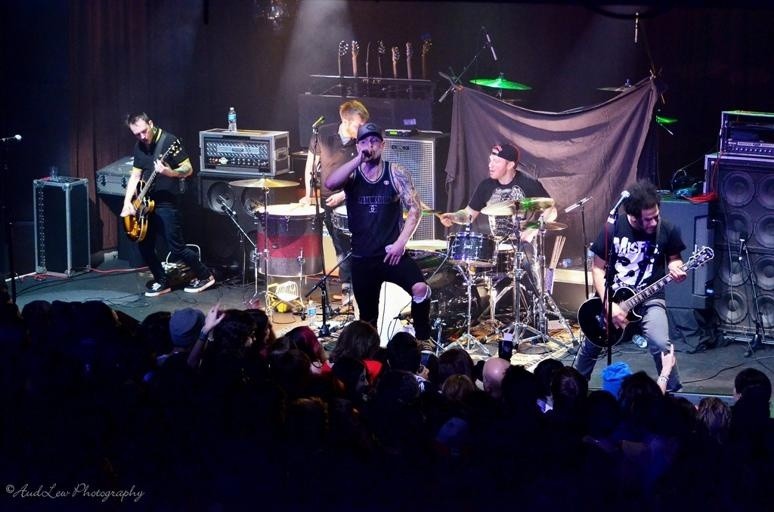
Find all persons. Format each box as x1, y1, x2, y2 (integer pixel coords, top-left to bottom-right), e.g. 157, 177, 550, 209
312, 319, 594, 512
325, 123, 434, 351
120, 110, 216, 297
297, 101, 369, 302
591, 358, 774, 510
0, 283, 324, 512
573, 181, 682, 394
439, 144, 558, 343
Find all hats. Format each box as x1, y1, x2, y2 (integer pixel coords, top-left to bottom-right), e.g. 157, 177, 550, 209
490, 142, 528, 169
170, 305, 206, 346
357, 122, 383, 144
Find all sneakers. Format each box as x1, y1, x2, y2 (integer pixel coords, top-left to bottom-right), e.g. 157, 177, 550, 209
416, 338, 436, 354
184, 275, 216, 293
340, 282, 355, 306
667, 384, 685, 393
144, 279, 172, 297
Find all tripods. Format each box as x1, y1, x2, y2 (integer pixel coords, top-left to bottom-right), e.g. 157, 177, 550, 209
494, 271, 577, 356
437, 286, 493, 357
248, 194, 302, 322
306, 254, 350, 339
510, 234, 581, 350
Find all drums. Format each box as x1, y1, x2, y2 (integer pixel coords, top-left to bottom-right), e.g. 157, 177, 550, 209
330, 205, 352, 259
447, 232, 497, 268
256, 205, 327, 278
476, 243, 518, 274
404, 249, 481, 354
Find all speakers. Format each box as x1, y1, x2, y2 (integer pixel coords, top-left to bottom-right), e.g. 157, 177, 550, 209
198, 171, 270, 277
702, 155, 774, 344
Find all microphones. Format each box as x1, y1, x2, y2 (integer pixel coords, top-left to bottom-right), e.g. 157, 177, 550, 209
362, 149, 373, 158
216, 194, 236, 218
1, 133, 23, 143
609, 190, 630, 215
312, 115, 327, 129
736, 230, 748, 260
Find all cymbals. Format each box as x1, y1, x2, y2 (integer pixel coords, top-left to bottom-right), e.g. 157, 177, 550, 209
403, 207, 437, 217
520, 221, 568, 232
481, 196, 555, 217
469, 72, 533, 91
596, 79, 634, 92
229, 177, 299, 190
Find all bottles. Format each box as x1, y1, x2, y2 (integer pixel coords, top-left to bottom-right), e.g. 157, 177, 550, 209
633, 334, 648, 348
555, 256, 581, 268
306, 300, 318, 326
587, 242, 596, 271
227, 107, 237, 132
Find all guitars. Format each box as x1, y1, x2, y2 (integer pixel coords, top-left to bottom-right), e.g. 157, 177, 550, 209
577, 247, 716, 348
349, 39, 362, 98
374, 39, 392, 99
402, 42, 414, 99
121, 138, 183, 243
391, 46, 403, 99
333, 40, 350, 96
418, 38, 435, 98
363, 40, 374, 97
